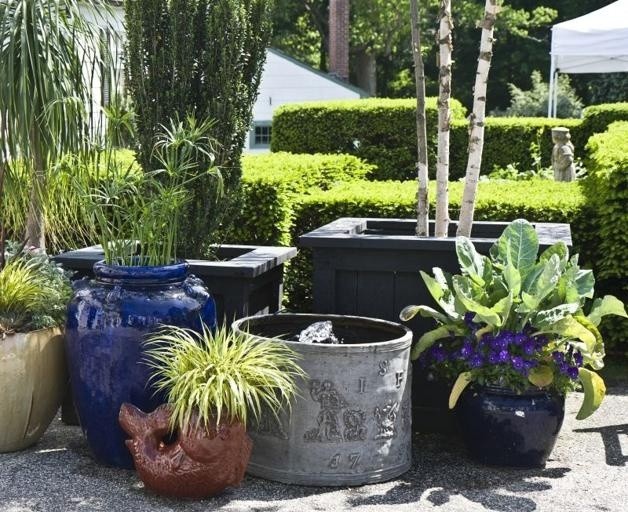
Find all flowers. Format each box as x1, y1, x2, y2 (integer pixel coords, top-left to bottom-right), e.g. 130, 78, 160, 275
392, 218, 628, 421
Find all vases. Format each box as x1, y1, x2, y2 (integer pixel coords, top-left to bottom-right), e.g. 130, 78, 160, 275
472, 382, 565, 468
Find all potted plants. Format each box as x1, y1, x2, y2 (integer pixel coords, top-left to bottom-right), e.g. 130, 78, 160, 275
0, 0, 301, 499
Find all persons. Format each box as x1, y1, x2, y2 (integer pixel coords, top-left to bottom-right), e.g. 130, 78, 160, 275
551, 128, 575, 182
